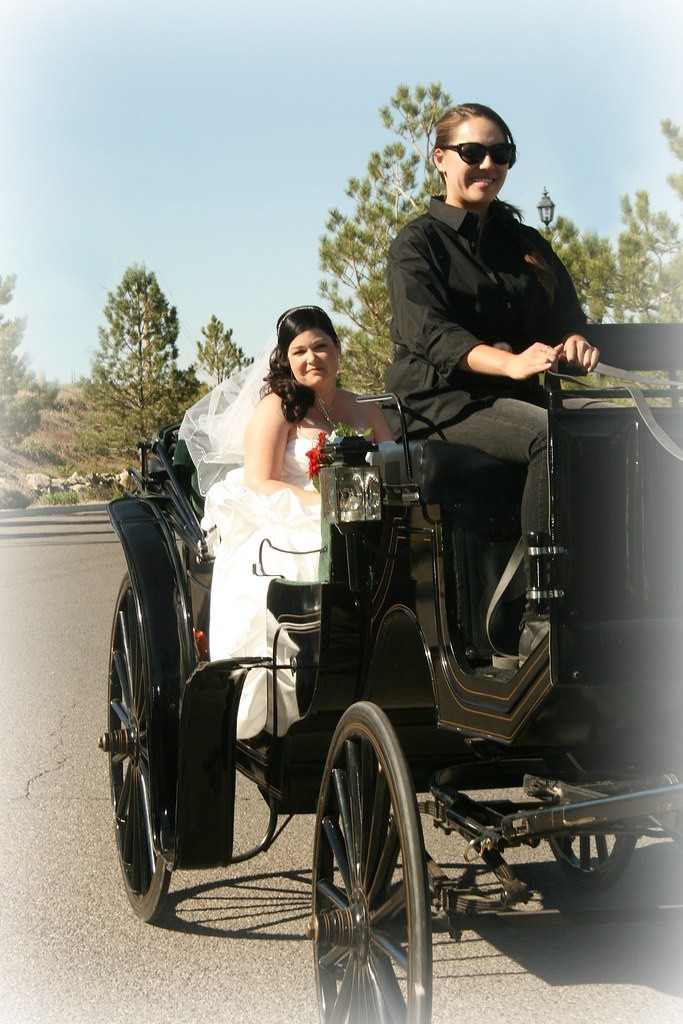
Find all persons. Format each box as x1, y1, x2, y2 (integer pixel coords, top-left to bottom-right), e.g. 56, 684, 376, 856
176, 304, 397, 739
388, 104, 599, 668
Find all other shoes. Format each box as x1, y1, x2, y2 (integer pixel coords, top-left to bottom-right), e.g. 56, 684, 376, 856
518, 622, 551, 669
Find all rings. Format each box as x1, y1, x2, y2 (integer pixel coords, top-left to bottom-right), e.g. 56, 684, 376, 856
545, 345, 550, 353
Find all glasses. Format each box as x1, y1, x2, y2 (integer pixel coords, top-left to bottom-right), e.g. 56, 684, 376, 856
438, 143, 516, 166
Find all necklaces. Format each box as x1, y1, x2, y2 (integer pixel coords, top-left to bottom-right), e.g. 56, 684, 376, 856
314, 402, 336, 422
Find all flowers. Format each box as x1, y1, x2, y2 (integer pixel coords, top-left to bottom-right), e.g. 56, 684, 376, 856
305, 421, 374, 494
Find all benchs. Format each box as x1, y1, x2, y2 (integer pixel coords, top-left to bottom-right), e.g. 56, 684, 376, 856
354, 324, 681, 663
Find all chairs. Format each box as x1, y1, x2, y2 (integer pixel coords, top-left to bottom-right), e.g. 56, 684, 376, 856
148, 422, 218, 662
